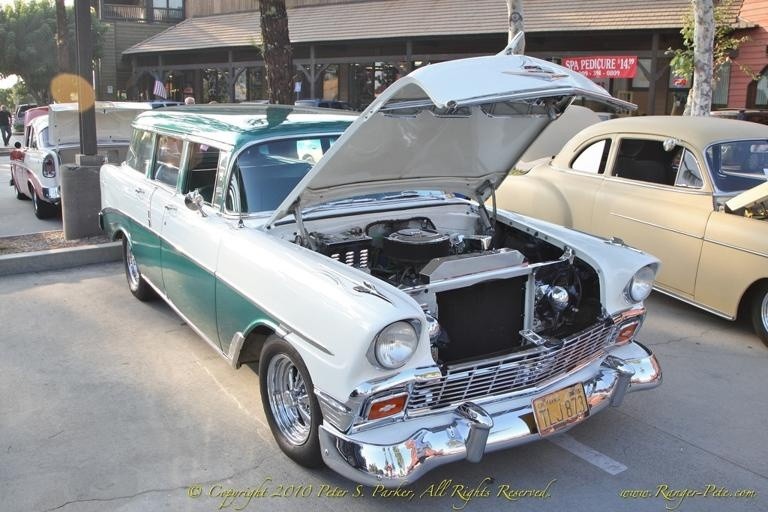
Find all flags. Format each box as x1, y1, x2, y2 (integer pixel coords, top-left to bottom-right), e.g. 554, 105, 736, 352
152, 79, 167, 100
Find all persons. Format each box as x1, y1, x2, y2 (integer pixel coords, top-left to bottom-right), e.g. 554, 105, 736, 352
185, 96, 195, 105
0, 104, 14, 146
669, 93, 685, 115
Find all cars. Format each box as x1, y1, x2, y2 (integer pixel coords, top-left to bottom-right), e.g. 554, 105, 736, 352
98, 27, 665, 491
477, 101, 768, 346
9, 97, 366, 220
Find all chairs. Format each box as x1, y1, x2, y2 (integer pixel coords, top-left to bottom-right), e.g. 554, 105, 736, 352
615, 141, 666, 182
188, 166, 218, 205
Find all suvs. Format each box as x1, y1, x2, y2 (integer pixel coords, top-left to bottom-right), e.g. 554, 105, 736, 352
706, 108, 768, 174
